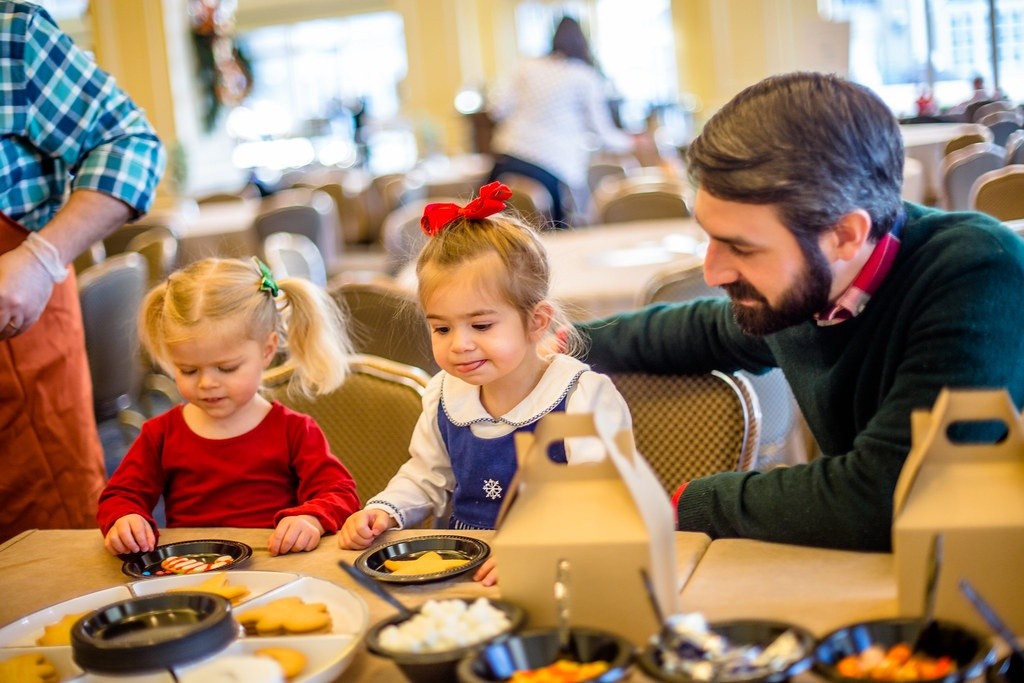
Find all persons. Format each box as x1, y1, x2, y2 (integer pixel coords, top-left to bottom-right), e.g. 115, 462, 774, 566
970, 77, 989, 101
529, 72, 1024, 554
340, 183, 640, 585
92, 257, 359, 554
489, 16, 654, 224
0, 0, 166, 530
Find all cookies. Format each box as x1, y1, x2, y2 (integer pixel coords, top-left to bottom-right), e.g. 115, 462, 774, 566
384, 550, 470, 576
507, 659, 609, 683
160, 556, 235, 573
0, 611, 95, 683
236, 596, 336, 678
165, 573, 250, 601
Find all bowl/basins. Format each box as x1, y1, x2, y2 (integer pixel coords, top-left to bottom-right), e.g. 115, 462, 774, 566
454, 624, 637, 682
806, 616, 994, 682
368, 590, 530, 682
634, 616, 822, 682
983, 639, 1024, 682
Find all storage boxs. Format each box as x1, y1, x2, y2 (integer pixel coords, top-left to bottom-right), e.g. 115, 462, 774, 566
890, 385, 1024, 642
490, 410, 668, 653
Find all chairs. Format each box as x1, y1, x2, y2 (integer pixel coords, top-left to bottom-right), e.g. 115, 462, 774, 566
72, 99, 1024, 529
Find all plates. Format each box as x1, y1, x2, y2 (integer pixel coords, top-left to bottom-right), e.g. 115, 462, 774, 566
355, 533, 492, 585
122, 539, 253, 579
0, 567, 369, 682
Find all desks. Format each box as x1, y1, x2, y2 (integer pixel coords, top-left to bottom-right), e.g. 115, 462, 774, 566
677, 536, 896, 639
534, 214, 708, 327
897, 122, 995, 201
0, 526, 715, 683
174, 197, 260, 268
